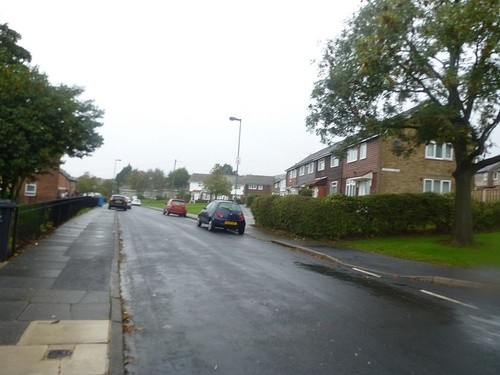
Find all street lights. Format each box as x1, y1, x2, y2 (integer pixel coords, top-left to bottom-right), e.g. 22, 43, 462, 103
230, 116, 242, 199
113, 159, 121, 194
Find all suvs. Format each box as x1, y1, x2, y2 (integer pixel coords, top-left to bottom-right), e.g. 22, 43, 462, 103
109, 195, 128, 211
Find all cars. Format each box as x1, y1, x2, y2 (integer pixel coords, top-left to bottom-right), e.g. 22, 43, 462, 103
197, 199, 246, 235
126, 196, 141, 209
163, 199, 187, 218
